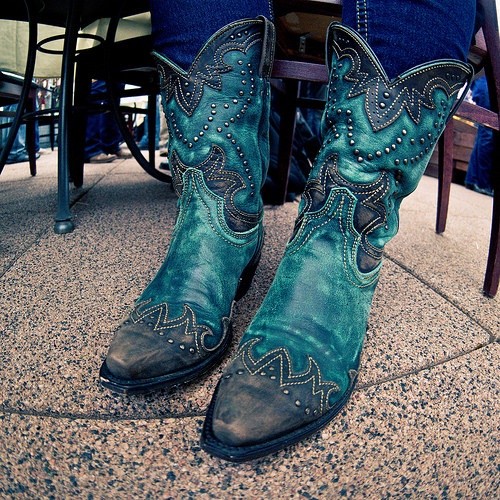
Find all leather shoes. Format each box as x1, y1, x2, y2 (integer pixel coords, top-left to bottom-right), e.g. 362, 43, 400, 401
6, 153, 41, 164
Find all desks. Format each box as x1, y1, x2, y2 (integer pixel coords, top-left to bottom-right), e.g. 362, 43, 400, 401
0, 0, 500, 301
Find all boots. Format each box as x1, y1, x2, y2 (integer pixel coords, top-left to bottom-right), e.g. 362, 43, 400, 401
97, 14, 279, 395
199, 23, 475, 463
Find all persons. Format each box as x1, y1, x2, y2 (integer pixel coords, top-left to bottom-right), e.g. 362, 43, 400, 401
98, 0, 478, 462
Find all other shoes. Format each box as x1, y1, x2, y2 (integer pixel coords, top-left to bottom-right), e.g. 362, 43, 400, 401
474, 185, 493, 196
464, 182, 474, 190
135, 135, 169, 170
87, 152, 117, 164
112, 149, 132, 159
